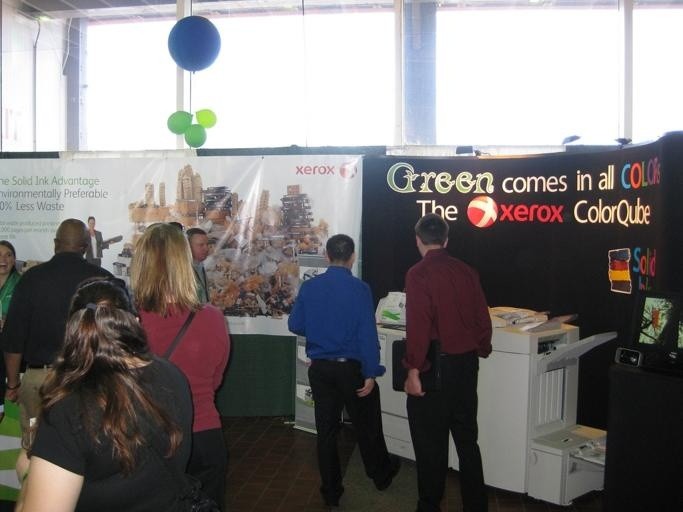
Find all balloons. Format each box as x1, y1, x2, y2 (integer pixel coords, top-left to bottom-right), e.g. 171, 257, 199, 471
168, 15, 221, 75
167, 111, 193, 134
196, 109, 217, 128
184, 124, 206, 148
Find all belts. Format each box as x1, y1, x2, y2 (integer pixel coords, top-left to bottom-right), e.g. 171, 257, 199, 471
28, 363, 53, 368
327, 357, 347, 362
440, 353, 464, 360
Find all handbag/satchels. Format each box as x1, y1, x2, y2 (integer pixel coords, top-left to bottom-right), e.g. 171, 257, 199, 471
169, 485, 219, 512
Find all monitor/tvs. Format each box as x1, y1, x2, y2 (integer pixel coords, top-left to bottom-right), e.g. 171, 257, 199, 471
630, 288, 682, 377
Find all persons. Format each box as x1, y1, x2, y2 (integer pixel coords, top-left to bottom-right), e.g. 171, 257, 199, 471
401, 212, 488, 511
288, 234, 392, 511
1, 218, 231, 511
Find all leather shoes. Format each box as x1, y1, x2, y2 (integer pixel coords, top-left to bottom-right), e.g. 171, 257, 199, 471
325, 486, 344, 505
374, 454, 399, 490
